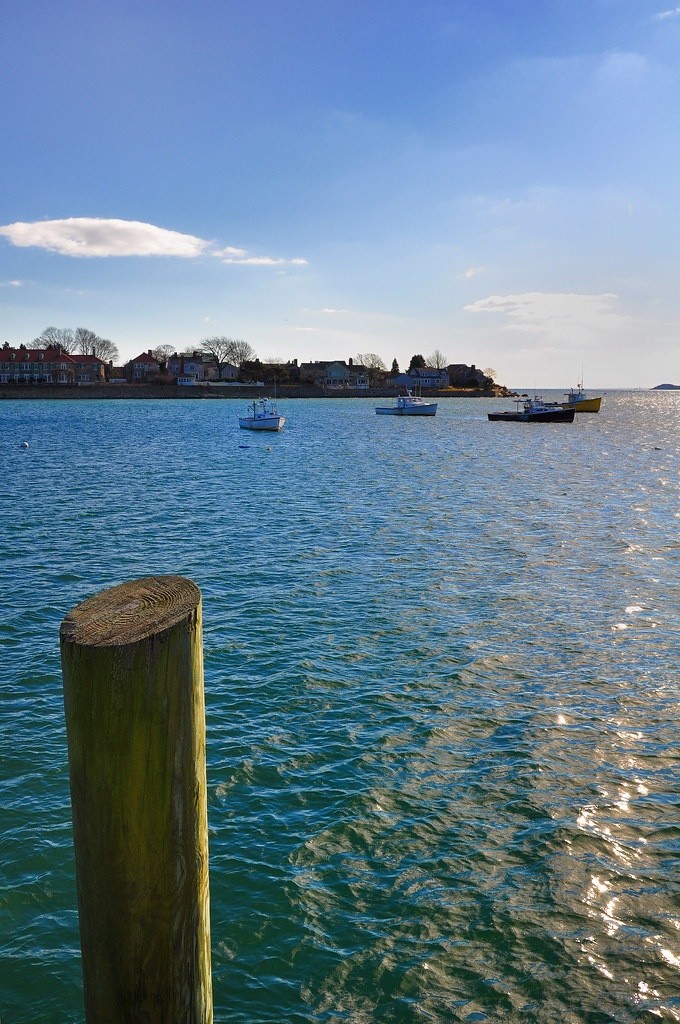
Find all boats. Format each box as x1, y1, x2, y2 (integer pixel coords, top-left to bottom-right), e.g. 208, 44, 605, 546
238, 379, 285, 431
486, 363, 602, 423
374, 380, 438, 417
